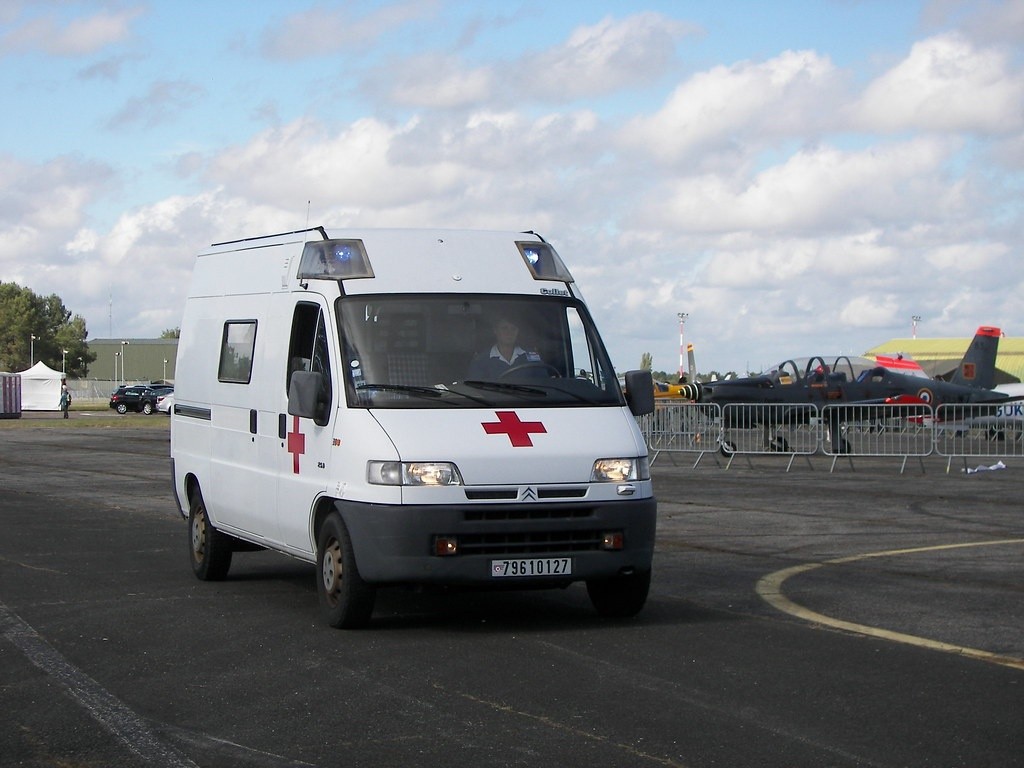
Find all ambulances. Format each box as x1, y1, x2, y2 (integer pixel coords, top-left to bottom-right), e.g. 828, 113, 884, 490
169, 223, 660, 629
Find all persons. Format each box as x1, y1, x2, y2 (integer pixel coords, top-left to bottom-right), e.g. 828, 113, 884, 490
467, 313, 563, 381
803, 365, 830, 387
58, 385, 72, 419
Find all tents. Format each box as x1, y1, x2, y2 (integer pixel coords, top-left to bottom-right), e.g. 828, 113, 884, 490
15, 360, 67, 411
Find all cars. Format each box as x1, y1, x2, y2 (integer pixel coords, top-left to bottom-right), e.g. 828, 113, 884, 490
154, 391, 174, 415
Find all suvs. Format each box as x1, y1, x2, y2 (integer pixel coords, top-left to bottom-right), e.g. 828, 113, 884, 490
109, 383, 174, 415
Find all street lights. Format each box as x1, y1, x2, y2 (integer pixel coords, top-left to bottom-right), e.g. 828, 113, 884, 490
30, 333, 40, 368
114, 351, 121, 388
163, 358, 168, 384
677, 312, 689, 382
120, 340, 130, 386
62, 349, 69, 373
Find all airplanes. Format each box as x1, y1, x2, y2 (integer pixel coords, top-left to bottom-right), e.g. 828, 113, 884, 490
863, 352, 1024, 441
652, 325, 1010, 457
596, 343, 704, 404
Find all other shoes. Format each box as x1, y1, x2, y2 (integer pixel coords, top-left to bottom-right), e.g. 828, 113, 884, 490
64, 416, 68, 419
